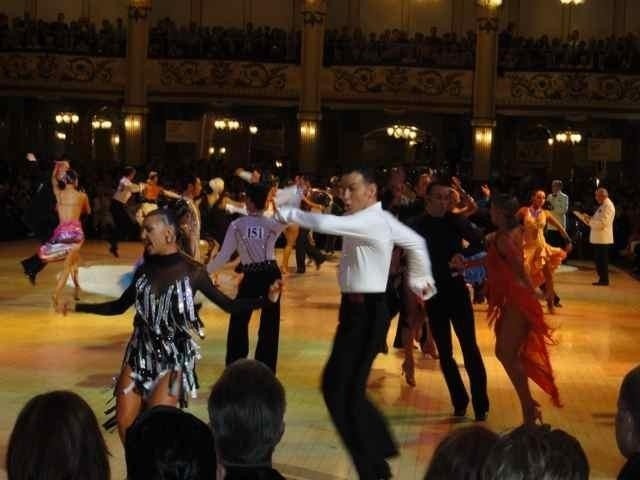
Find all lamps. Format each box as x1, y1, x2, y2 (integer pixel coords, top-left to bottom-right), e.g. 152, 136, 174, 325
53, 114, 80, 122
91, 118, 112, 129
548, 130, 581, 146
386, 125, 421, 140
214, 117, 238, 130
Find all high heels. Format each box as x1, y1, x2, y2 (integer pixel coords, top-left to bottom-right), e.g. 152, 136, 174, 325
423, 344, 440, 360
524, 400, 544, 426
402, 362, 416, 386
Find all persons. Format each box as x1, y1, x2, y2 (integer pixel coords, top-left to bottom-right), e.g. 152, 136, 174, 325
276, 158, 436, 479
7, 359, 287, 479
109, 166, 346, 273
425, 366, 639, 479
57, 207, 283, 451
0, 9, 127, 57
324, 22, 477, 70
381, 165, 615, 424
148, 16, 302, 64
497, 20, 640, 75
204, 186, 288, 374
21, 158, 92, 309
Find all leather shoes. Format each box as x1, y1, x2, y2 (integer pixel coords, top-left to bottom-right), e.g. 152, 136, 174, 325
454, 399, 488, 421
